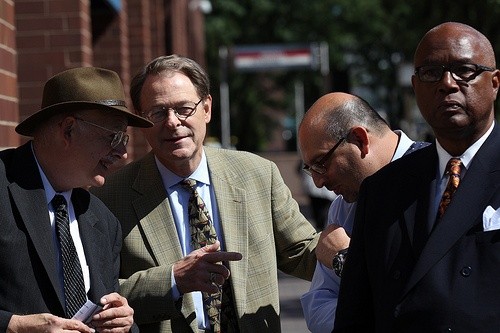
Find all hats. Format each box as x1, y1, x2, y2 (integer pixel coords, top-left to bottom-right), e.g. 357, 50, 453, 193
14, 66, 155, 139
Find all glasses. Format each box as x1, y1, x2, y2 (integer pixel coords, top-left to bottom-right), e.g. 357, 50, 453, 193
413, 61, 495, 84
139, 96, 204, 126
57, 115, 130, 150
302, 126, 369, 178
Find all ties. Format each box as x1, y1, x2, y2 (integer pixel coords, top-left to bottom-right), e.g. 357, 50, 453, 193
180, 179, 231, 333
50, 194, 88, 320
433, 157, 463, 229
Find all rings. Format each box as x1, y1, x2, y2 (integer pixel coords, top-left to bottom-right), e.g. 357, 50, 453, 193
210, 272, 217, 282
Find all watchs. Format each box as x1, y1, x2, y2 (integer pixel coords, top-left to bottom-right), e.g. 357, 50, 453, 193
332, 247, 349, 278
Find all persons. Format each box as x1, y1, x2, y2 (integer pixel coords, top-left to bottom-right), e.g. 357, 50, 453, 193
89, 53, 323, 333
331, 22, 500, 333
0, 66, 155, 333
297, 92, 432, 333
